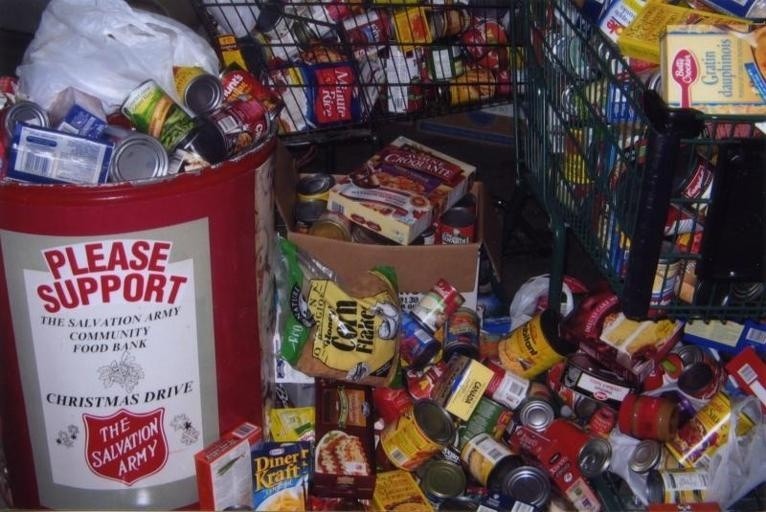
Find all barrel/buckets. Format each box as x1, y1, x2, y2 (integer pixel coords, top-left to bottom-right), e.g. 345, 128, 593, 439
0, 97, 281, 510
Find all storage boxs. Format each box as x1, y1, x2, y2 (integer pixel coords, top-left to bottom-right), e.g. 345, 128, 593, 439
617, 1, 765, 117
268, 140, 505, 389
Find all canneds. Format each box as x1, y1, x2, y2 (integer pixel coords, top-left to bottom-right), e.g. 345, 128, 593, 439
0, 0, 766, 512
